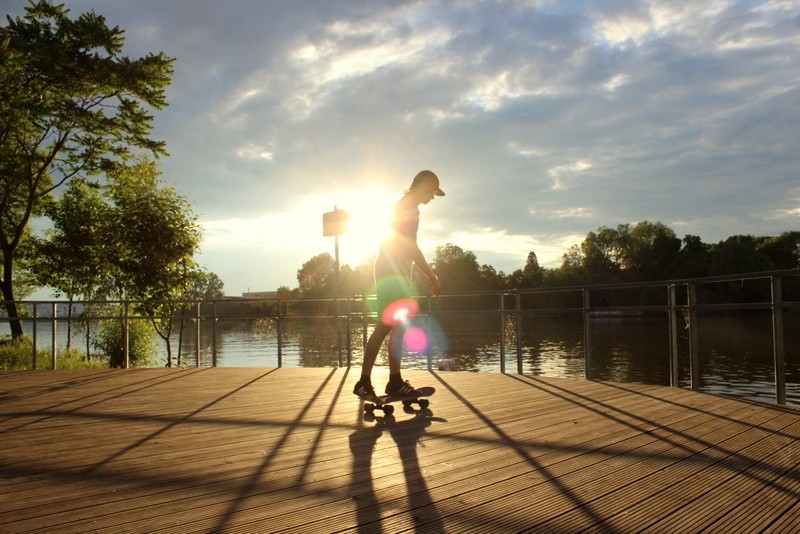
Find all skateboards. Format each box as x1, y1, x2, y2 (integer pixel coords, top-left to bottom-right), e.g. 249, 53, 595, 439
357, 387, 435, 415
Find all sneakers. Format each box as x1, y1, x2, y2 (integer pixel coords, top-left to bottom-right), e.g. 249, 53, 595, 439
385, 379, 424, 396
353, 381, 383, 403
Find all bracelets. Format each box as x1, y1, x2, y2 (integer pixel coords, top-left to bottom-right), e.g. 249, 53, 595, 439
430, 275, 438, 283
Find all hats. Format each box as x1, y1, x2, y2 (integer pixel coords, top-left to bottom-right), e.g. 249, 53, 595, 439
414, 170, 445, 196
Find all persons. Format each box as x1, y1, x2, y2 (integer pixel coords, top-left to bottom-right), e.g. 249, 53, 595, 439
353, 170, 446, 402
